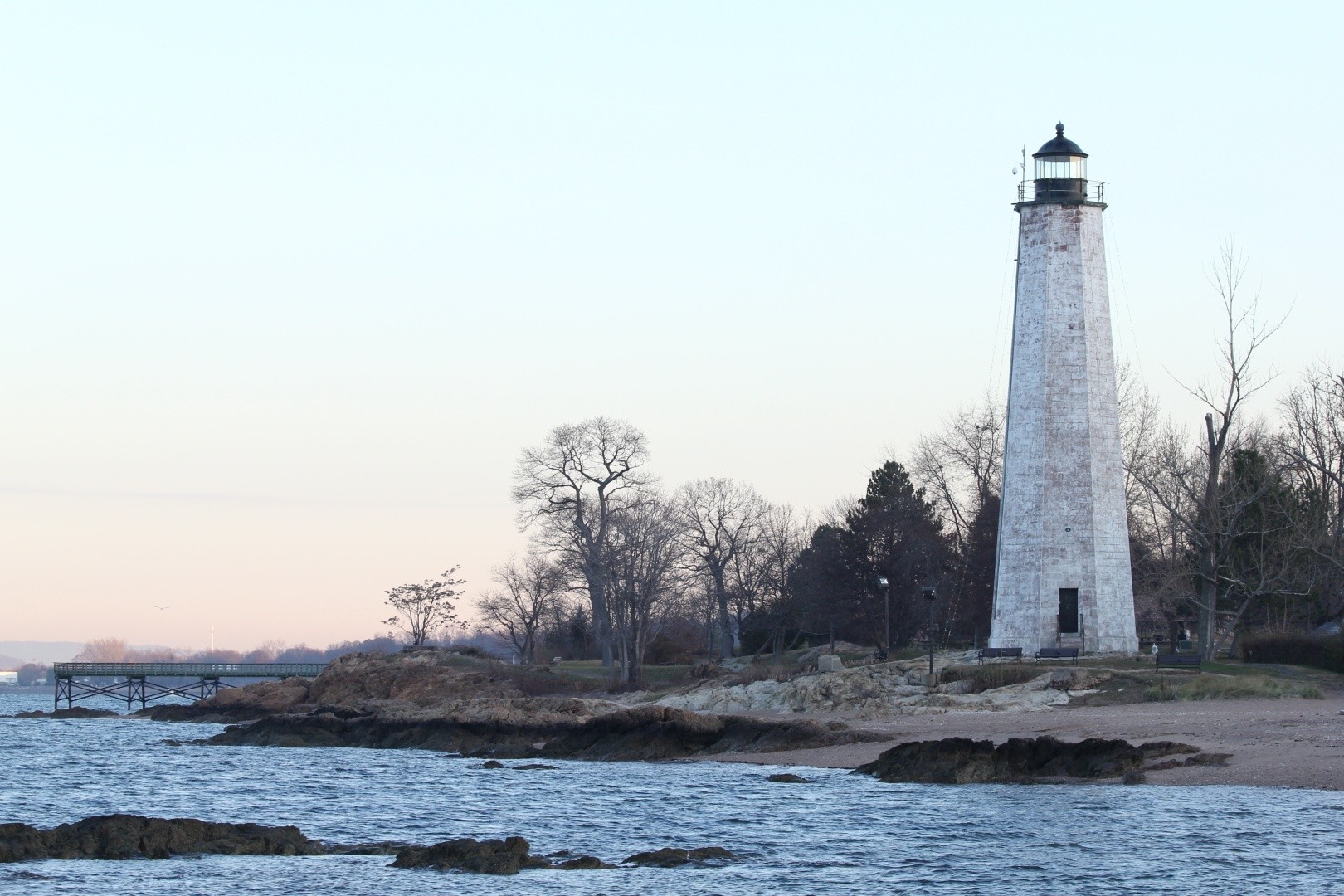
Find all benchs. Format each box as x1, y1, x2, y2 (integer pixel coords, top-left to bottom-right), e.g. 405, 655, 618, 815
873, 650, 888, 663
978, 647, 1023, 664
1156, 654, 1203, 674
660, 657, 678, 666
1035, 647, 1079, 666
1177, 641, 1194, 654
1219, 648, 1230, 654
550, 657, 562, 663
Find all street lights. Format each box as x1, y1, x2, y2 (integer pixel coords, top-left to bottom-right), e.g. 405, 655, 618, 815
921, 586, 937, 687
879, 577, 890, 662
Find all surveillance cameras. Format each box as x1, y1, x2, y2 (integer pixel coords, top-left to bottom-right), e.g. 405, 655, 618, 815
1012, 168, 1017, 174
